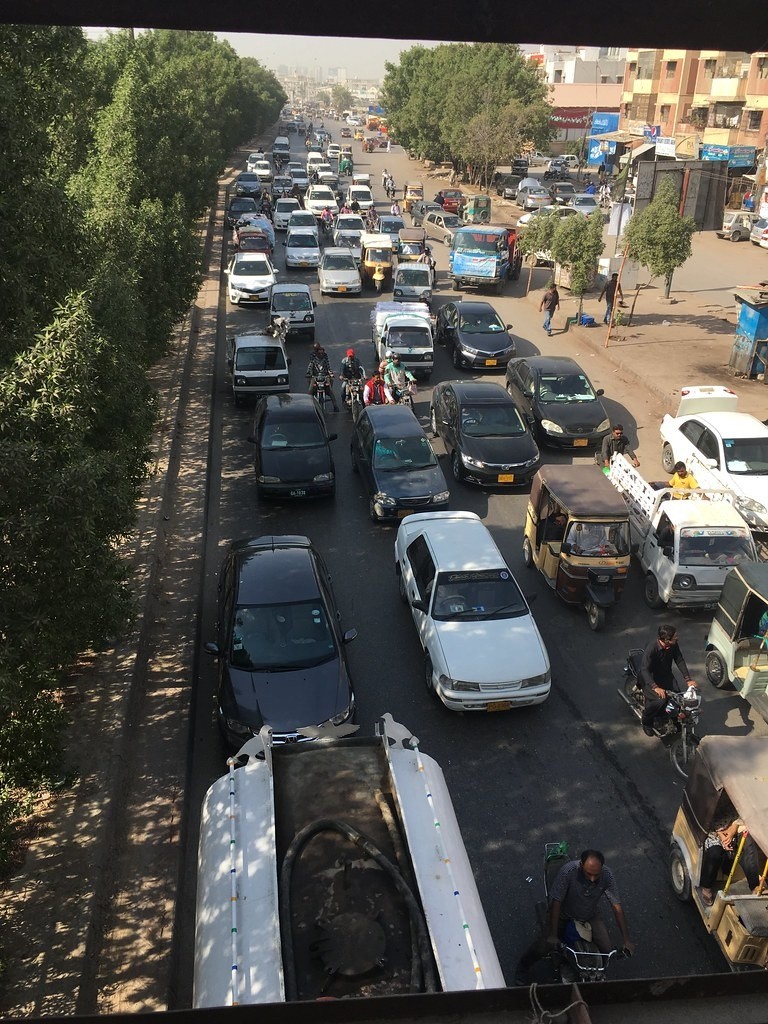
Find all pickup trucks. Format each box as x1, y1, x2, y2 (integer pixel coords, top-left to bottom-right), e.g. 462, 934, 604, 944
369, 302, 435, 381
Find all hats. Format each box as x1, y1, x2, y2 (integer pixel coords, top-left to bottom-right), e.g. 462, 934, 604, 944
347, 349, 353, 357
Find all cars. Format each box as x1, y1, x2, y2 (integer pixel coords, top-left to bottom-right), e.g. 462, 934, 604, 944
351, 405, 451, 521
247, 393, 338, 502
716, 212, 768, 249
394, 511, 552, 713
224, 106, 602, 405
430, 379, 540, 486
216, 535, 356, 759
661, 385, 768, 533
505, 355, 610, 450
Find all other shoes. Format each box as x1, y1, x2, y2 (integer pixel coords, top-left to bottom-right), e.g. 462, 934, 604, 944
644, 724, 654, 736
543, 325, 546, 330
700, 887, 714, 905
547, 331, 551, 336
752, 885, 768, 895
334, 407, 339, 412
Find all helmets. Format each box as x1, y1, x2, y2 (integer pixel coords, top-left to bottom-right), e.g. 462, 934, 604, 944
391, 352, 401, 360
386, 351, 392, 358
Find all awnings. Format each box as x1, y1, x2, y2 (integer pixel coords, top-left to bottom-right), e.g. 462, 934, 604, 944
619, 143, 655, 164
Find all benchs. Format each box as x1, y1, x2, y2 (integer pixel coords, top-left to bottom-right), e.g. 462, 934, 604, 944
543, 542, 561, 580
734, 637, 768, 667
716, 899, 768, 964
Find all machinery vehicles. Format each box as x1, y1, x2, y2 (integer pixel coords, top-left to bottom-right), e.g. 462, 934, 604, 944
193, 713, 507, 1005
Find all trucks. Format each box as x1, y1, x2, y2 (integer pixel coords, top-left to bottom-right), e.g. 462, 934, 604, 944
447, 223, 523, 297
607, 453, 759, 611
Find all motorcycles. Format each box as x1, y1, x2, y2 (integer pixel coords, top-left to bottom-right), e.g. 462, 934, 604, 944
310, 375, 417, 422
383, 177, 396, 202
618, 649, 703, 780
457, 194, 492, 224
536, 840, 631, 981
524, 465, 641, 632
544, 169, 560, 182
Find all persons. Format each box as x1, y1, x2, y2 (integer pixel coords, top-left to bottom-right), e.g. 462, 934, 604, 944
258, 134, 355, 183
433, 187, 474, 219
292, 183, 301, 198
640, 624, 698, 737
260, 188, 272, 206
664, 461, 710, 500
657, 523, 676, 556
321, 205, 335, 234
700, 795, 768, 905
539, 283, 562, 336
425, 577, 450, 598
566, 522, 608, 556
547, 849, 637, 982
599, 272, 624, 328
382, 169, 396, 197
340, 198, 381, 227
601, 423, 640, 469
416, 247, 436, 289
309, 347, 417, 413
392, 200, 401, 217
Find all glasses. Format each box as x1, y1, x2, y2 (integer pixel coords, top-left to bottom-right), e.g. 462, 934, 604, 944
614, 432, 622, 435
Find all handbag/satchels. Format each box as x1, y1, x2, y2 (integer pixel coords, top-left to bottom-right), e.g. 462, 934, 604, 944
684, 685, 701, 725
406, 380, 417, 396
